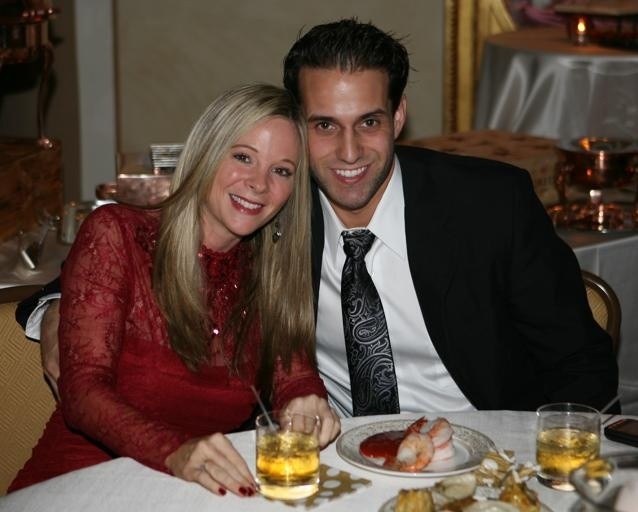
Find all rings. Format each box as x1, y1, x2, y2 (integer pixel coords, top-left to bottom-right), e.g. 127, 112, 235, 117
313, 415, 322, 423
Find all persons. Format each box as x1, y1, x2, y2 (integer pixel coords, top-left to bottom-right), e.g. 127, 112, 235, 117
5, 84, 342, 495
283, 21, 622, 419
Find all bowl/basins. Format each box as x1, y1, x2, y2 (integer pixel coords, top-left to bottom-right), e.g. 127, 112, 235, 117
568, 450, 637, 512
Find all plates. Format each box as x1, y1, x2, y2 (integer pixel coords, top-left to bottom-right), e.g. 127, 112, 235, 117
336, 420, 495, 477
378, 484, 551, 511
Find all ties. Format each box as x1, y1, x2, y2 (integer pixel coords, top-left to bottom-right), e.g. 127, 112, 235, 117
341, 230, 400, 415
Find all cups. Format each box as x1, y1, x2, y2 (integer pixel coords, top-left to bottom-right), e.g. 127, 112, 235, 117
255, 411, 321, 499
533, 401, 602, 495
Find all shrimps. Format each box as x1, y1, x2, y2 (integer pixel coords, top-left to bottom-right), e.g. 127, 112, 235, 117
396, 432, 433, 472
404, 415, 455, 461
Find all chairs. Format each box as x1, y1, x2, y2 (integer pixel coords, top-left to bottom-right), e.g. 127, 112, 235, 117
582, 270, 621, 342
1, 284, 58, 495
